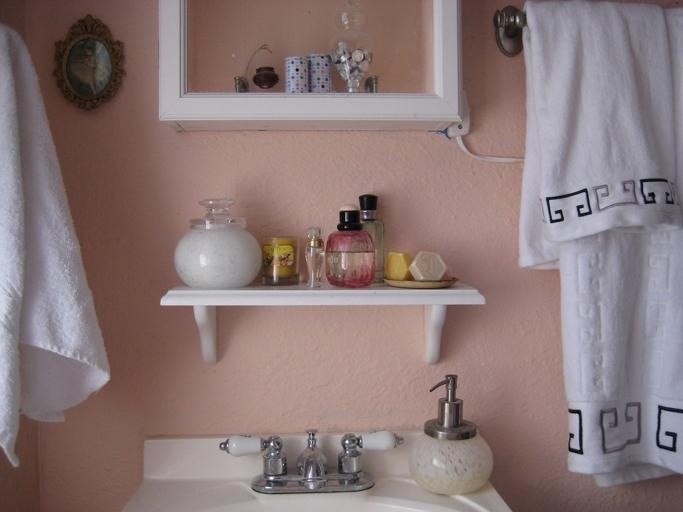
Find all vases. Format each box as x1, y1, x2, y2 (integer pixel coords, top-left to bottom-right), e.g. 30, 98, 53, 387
175, 199, 264, 288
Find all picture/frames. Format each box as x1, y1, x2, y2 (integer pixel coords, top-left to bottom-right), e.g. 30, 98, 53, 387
50, 12, 127, 112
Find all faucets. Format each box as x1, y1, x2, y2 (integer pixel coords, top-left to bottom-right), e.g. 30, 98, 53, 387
294, 427, 326, 491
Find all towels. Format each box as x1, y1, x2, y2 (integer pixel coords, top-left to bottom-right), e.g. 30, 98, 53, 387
518, 0, 683, 490
0, 21, 112, 467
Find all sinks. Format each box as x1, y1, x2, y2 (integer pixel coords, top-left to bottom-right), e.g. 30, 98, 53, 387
211, 492, 492, 512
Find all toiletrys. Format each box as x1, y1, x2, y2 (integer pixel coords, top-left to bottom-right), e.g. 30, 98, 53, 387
305, 224, 327, 289
323, 204, 377, 288
356, 192, 385, 283
